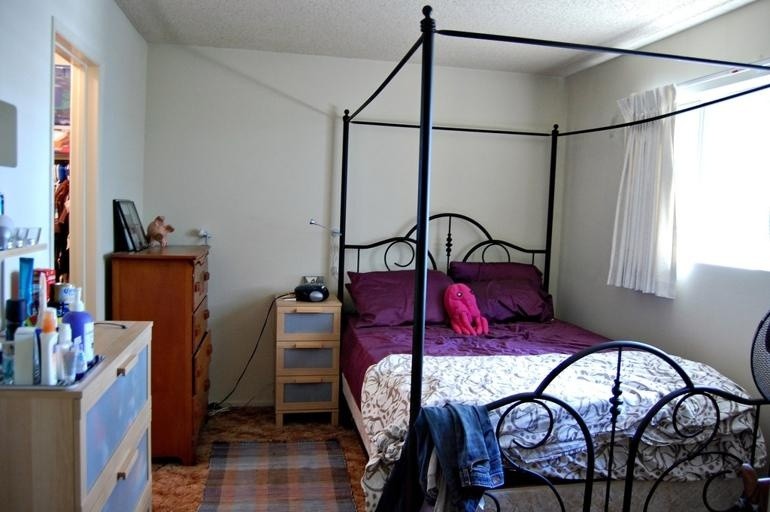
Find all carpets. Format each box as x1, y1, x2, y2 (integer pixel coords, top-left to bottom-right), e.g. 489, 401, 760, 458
198, 439, 357, 512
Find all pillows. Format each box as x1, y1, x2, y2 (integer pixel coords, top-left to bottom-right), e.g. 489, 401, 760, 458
346, 261, 554, 328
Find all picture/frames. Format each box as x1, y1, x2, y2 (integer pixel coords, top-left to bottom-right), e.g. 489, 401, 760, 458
114, 199, 149, 251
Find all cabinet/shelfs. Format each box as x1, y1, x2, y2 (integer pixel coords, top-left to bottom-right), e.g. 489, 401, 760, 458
0, 321, 154, 511
110, 246, 213, 466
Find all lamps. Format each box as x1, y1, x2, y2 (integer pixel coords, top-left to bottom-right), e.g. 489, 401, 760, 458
310, 219, 342, 236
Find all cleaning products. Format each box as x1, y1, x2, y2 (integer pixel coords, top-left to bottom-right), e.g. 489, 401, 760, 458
36, 290, 95, 387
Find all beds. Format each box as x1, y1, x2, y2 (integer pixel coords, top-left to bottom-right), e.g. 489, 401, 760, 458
337, 6, 770, 512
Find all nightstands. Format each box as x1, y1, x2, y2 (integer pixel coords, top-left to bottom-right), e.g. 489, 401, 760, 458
275, 295, 342, 429
